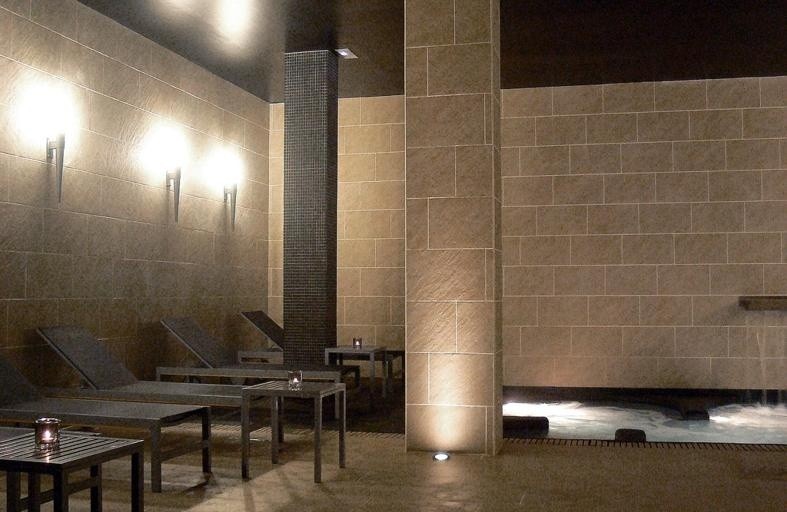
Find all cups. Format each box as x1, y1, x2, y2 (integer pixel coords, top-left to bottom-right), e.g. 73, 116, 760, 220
33, 417, 62, 451
287, 370, 303, 391
352, 336, 362, 349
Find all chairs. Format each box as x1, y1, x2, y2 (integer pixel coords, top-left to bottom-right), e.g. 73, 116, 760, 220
161, 316, 361, 410
0, 357, 211, 492
241, 308, 405, 399
37, 323, 287, 480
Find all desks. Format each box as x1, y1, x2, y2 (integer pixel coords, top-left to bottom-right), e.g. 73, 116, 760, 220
240, 380, 349, 484
0, 431, 142, 512
324, 344, 389, 398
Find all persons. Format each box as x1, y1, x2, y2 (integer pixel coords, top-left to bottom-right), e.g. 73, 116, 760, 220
680, 400, 711, 421
